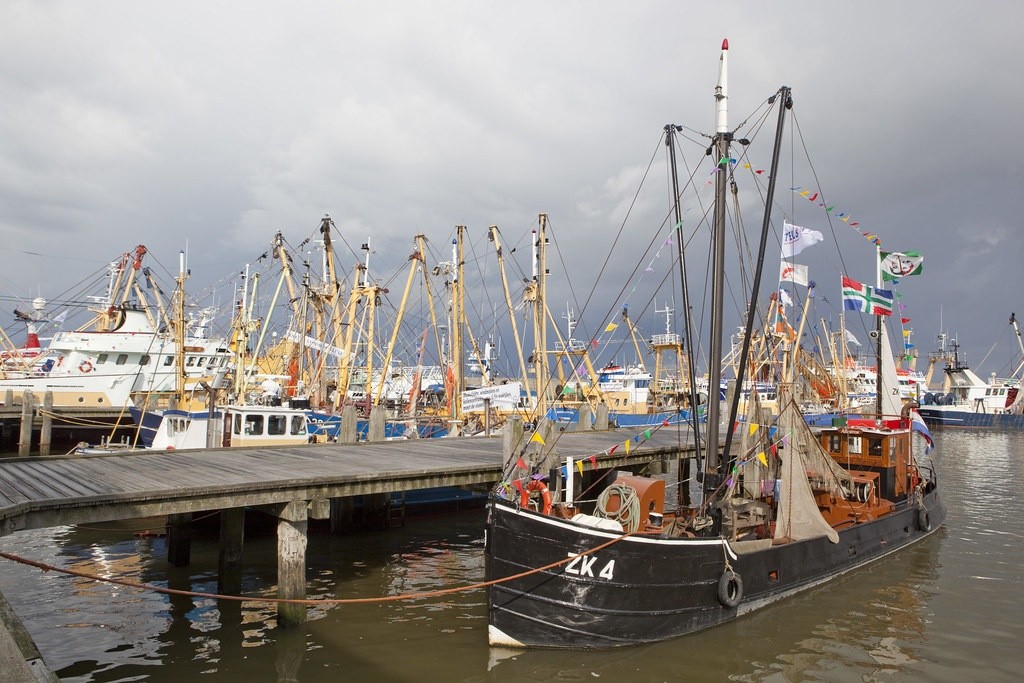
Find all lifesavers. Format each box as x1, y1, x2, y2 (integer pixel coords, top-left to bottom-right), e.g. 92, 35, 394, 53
78, 360, 93, 374
918, 507, 933, 532
520, 480, 552, 516
718, 571, 743, 609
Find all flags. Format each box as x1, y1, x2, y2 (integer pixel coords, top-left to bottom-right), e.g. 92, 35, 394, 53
879, 250, 924, 281
777, 290, 798, 345
54, 310, 69, 323
782, 224, 823, 258
842, 276, 893, 315
781, 261, 808, 287
911, 408, 934, 455
844, 329, 860, 346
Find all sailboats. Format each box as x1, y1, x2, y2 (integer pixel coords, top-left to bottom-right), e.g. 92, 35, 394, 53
2, 167, 1024, 459
481, 37, 950, 649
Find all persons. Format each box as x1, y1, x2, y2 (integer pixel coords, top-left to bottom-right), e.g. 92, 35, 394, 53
262, 378, 282, 406
772, 473, 782, 523
